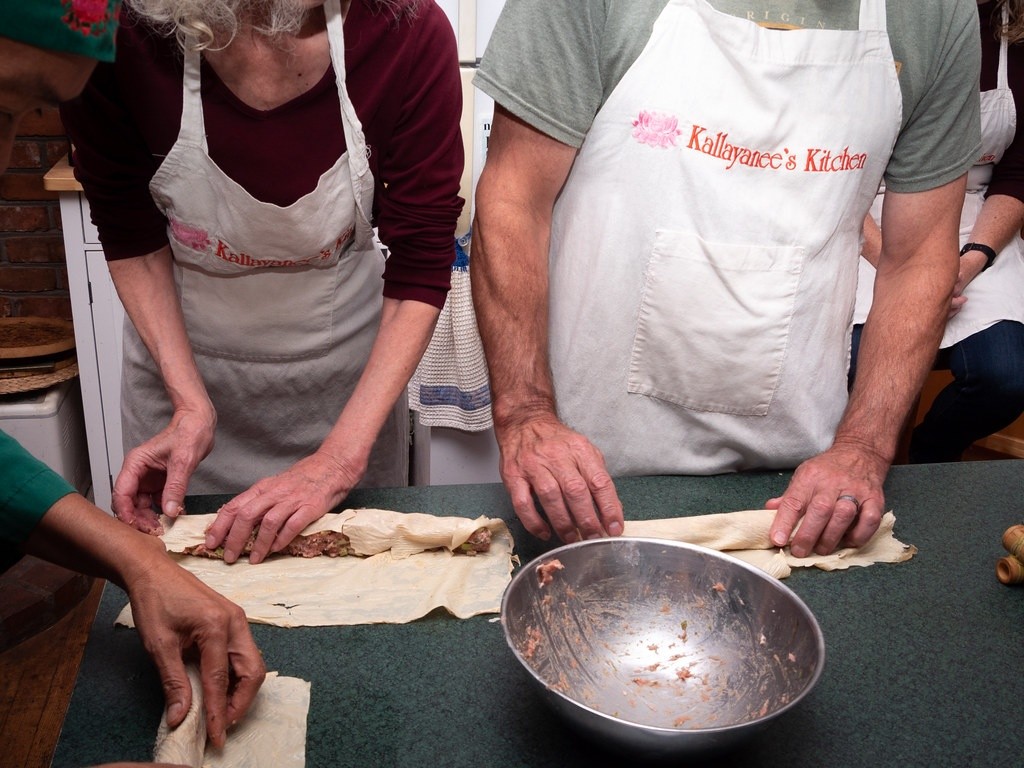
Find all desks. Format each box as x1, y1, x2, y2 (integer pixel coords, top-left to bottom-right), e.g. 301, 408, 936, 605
47, 458, 1023, 768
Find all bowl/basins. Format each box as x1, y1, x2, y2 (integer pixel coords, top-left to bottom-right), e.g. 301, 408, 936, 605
501, 537, 826, 763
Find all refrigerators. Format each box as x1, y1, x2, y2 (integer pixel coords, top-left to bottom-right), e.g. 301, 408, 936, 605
414, 0, 503, 486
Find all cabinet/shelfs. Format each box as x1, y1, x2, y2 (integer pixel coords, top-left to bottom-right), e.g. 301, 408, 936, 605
44, 150, 126, 515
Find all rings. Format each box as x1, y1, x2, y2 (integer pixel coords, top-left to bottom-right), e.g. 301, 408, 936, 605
836, 495, 859, 510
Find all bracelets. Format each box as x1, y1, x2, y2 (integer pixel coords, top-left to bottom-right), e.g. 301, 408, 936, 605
960, 242, 996, 272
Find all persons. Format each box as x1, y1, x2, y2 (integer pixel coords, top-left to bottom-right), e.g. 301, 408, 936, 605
848, 0, 1024, 464
60, 0, 464, 564
471, 0, 984, 558
0, 0, 266, 749
